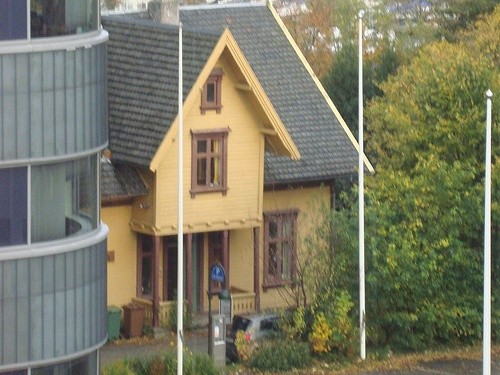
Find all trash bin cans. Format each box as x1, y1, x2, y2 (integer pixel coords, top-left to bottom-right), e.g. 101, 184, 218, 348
123, 303, 145, 339
105, 304, 122, 343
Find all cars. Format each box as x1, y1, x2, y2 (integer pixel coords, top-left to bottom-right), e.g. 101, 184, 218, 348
226, 312, 282, 363
